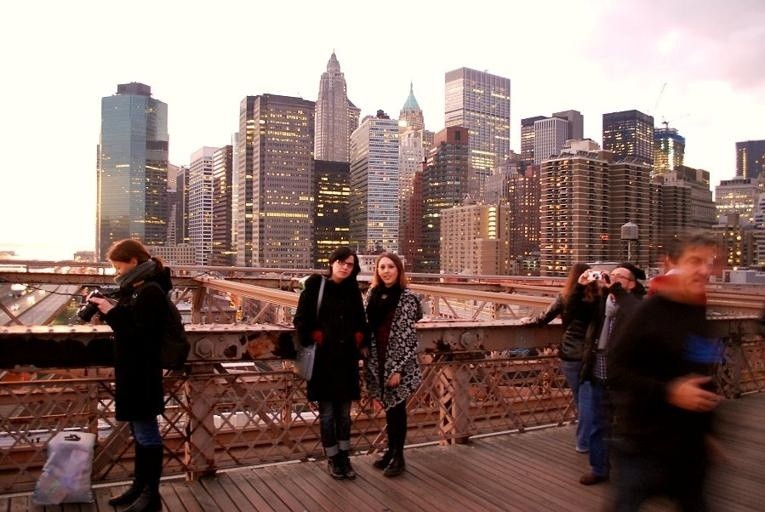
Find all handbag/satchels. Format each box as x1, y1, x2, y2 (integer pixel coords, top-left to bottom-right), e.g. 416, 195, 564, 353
31, 432, 95, 505
293, 275, 325, 381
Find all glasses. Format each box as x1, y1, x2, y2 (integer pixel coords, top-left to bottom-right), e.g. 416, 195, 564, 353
611, 273, 635, 282
335, 258, 355, 269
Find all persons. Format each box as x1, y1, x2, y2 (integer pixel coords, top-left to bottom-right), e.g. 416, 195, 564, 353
294, 247, 363, 481
578, 263, 646, 485
522, 262, 600, 453
363, 253, 422, 478
89, 240, 189, 512
605, 233, 725, 512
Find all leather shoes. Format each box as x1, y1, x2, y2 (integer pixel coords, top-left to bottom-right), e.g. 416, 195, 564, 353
383, 456, 405, 476
374, 453, 390, 467
580, 473, 602, 484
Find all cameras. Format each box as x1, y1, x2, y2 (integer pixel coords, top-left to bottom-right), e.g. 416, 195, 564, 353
77, 289, 105, 322
587, 271, 604, 282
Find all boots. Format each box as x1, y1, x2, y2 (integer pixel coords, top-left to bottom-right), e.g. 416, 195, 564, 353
122, 481, 162, 512
108, 477, 144, 506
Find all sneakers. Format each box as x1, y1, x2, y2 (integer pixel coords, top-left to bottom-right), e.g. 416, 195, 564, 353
342, 454, 355, 479
575, 444, 589, 452
327, 456, 344, 479
